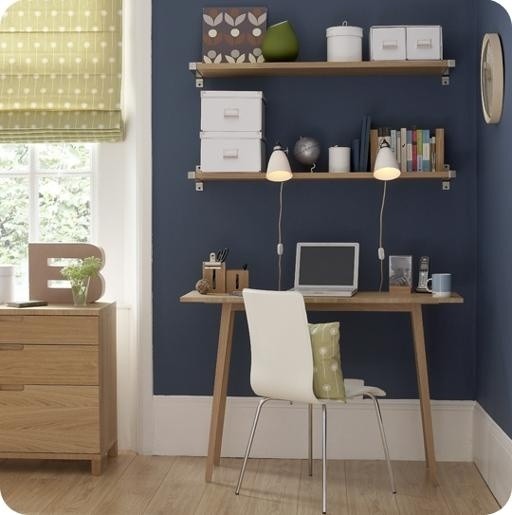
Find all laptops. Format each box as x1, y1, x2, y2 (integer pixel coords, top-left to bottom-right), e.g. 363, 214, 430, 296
286, 242, 360, 297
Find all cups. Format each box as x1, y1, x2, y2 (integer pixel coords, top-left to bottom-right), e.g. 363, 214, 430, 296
327, 146, 350, 173
426, 273, 453, 298
1, 265, 16, 305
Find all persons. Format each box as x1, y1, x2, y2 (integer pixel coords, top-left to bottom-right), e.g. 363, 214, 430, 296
389, 256, 410, 286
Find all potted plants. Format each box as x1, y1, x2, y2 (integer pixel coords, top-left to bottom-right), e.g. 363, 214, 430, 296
60, 252, 103, 306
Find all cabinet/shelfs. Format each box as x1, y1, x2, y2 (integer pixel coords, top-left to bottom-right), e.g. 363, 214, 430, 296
185, 54, 461, 192
1, 301, 121, 476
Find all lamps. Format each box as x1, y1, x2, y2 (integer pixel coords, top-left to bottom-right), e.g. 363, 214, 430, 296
260, 142, 297, 290
371, 139, 404, 294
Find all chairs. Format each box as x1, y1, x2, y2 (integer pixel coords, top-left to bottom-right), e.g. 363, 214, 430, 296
234, 285, 400, 515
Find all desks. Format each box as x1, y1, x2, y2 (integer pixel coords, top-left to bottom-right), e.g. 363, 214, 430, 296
177, 287, 465, 484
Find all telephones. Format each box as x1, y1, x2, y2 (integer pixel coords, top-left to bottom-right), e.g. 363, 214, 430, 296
413, 255, 432, 293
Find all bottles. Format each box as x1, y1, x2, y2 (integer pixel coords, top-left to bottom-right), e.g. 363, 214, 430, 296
325, 20, 367, 61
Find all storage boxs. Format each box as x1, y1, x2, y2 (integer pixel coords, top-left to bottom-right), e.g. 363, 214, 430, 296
197, 131, 268, 174
198, 87, 266, 133
368, 24, 406, 61
406, 24, 447, 62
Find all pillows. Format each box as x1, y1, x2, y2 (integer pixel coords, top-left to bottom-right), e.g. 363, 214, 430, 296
305, 320, 349, 406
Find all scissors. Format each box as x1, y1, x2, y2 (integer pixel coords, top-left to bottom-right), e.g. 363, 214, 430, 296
215, 248, 229, 263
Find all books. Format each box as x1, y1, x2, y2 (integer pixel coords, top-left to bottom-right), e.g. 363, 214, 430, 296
353, 112, 446, 172
